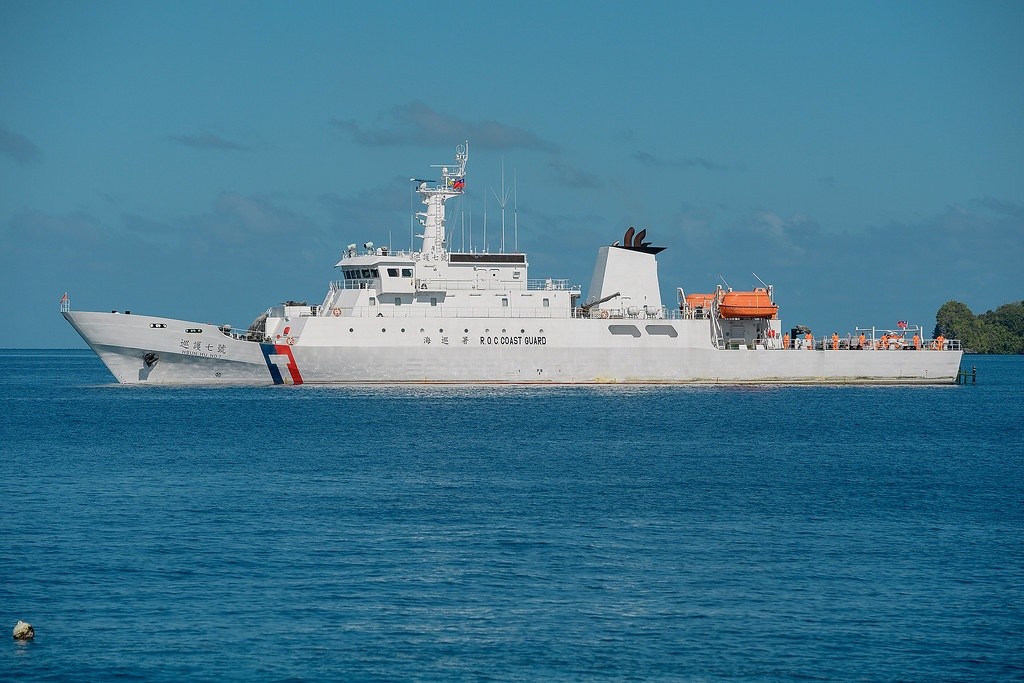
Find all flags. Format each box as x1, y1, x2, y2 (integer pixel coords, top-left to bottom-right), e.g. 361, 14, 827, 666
453, 179, 465, 189
897, 320, 907, 328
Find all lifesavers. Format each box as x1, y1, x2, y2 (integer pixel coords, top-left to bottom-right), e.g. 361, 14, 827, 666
332, 307, 342, 317
601, 311, 609, 319
286, 337, 295, 345
930, 342, 938, 350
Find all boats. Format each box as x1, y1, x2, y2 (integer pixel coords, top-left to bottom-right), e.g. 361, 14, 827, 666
62, 139, 977, 383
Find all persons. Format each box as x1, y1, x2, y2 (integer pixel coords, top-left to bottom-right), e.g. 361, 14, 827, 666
783, 331, 944, 351
